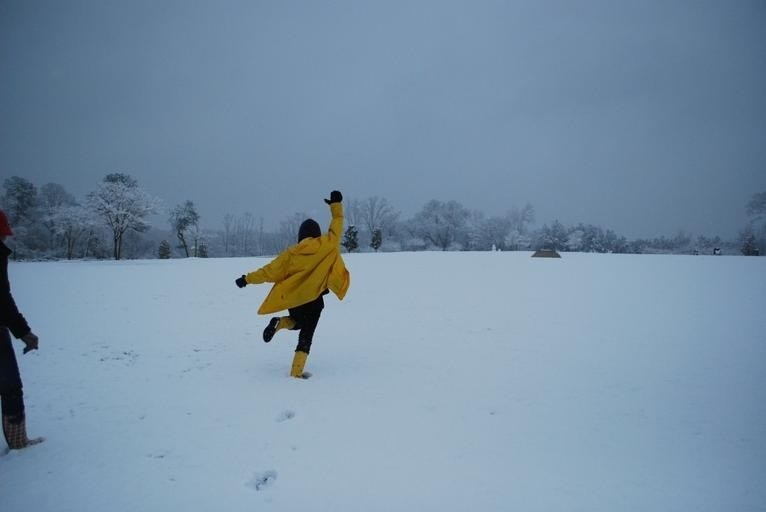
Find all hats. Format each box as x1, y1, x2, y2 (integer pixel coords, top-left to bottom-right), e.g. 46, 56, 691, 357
298, 219, 321, 244
0, 209, 13, 235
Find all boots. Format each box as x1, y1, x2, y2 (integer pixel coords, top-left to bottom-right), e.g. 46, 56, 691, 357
289, 350, 309, 379
2, 415, 45, 449
263, 316, 295, 343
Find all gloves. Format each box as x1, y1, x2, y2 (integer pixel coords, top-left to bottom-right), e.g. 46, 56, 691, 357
235, 275, 247, 288
323, 191, 343, 205
21, 331, 39, 355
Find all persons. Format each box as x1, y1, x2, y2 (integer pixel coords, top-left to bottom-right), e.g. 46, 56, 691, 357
236, 191, 351, 379
0, 209, 45, 449
